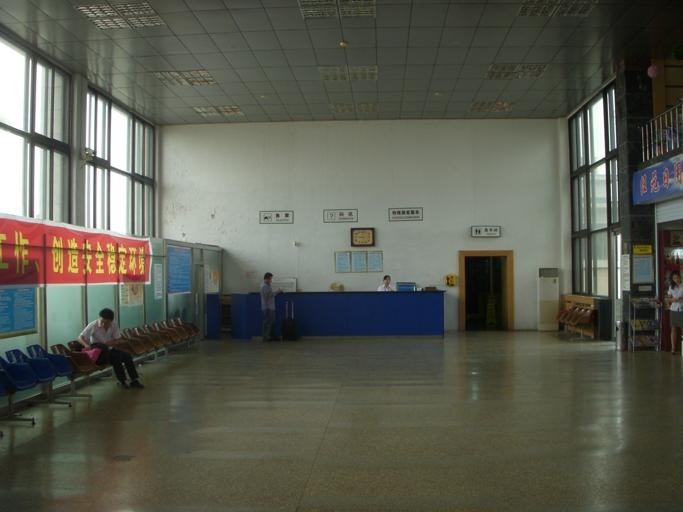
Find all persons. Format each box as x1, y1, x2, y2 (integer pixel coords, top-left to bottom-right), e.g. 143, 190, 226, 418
77, 308, 145, 389
260, 272, 283, 341
376, 274, 393, 292
666, 269, 683, 355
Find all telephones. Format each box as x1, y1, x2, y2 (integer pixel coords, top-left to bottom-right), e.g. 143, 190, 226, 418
444, 274, 457, 287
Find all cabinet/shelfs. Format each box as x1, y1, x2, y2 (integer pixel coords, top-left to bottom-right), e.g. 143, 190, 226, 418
628, 295, 662, 353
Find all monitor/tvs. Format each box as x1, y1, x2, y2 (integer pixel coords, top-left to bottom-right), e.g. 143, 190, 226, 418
396, 281, 416, 292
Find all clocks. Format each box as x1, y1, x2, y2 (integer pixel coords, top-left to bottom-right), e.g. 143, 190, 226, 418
350, 227, 374, 246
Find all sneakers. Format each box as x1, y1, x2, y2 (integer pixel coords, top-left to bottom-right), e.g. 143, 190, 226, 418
129, 380, 144, 389
117, 382, 129, 389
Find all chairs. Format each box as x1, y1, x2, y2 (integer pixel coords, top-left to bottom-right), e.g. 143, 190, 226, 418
556, 305, 593, 341
0, 318, 197, 434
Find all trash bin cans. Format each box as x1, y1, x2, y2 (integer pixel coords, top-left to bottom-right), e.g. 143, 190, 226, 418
615, 321, 628, 351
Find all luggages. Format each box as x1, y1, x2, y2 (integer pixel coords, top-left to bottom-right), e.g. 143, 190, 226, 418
280, 301, 297, 342
